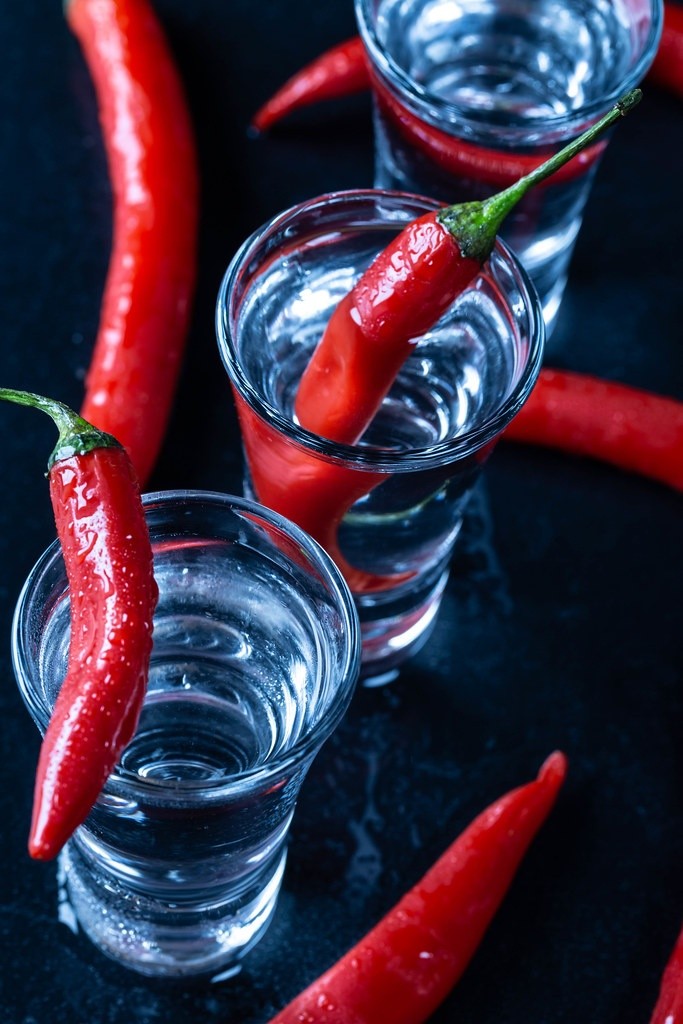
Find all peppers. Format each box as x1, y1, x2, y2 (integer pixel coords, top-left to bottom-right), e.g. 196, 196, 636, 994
1, 0, 683, 1024
